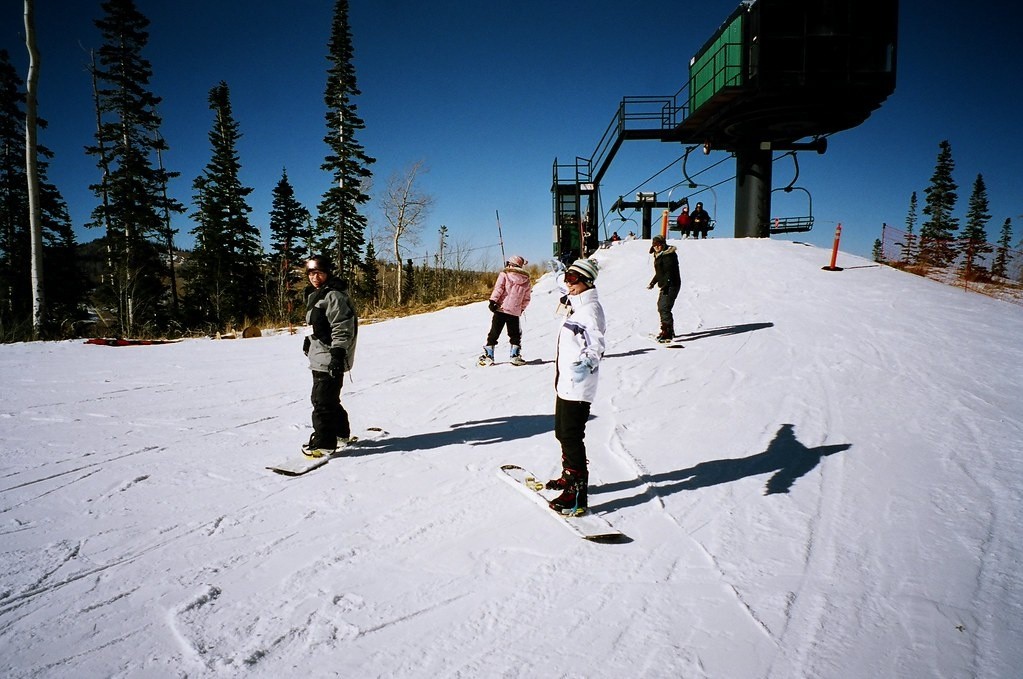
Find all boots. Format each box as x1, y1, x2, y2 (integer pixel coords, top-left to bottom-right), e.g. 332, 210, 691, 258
479, 346, 494, 366
549, 462, 588, 515
545, 455, 568, 490
510, 344, 526, 365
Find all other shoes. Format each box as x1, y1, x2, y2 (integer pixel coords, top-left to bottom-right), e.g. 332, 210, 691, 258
657, 327, 675, 339
659, 333, 672, 342
572, 357, 592, 381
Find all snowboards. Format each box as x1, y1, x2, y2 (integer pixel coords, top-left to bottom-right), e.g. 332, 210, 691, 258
639, 330, 684, 348
264, 427, 385, 476
458, 358, 544, 371
499, 464, 626, 543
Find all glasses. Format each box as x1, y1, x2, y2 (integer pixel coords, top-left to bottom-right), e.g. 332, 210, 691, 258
564, 272, 579, 284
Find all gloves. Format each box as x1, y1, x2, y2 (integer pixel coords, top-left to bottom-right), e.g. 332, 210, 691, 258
489, 300, 496, 312
303, 336, 311, 356
328, 347, 346, 380
648, 283, 654, 289
659, 283, 670, 296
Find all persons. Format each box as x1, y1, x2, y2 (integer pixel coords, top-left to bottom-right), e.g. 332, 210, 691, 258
582, 212, 591, 254
476, 256, 531, 366
553, 252, 566, 272
626, 232, 635, 240
564, 214, 576, 223
302, 256, 358, 457
677, 207, 690, 239
610, 232, 621, 241
690, 202, 711, 240
646, 235, 681, 343
546, 258, 605, 515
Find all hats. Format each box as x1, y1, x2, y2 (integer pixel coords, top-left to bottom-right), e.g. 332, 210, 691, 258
567, 258, 601, 288
508, 255, 528, 268
649, 235, 668, 254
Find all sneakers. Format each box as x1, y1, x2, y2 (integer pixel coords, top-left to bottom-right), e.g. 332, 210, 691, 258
302, 445, 335, 457
336, 436, 349, 447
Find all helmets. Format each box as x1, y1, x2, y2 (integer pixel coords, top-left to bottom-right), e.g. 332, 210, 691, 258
305, 255, 330, 276
